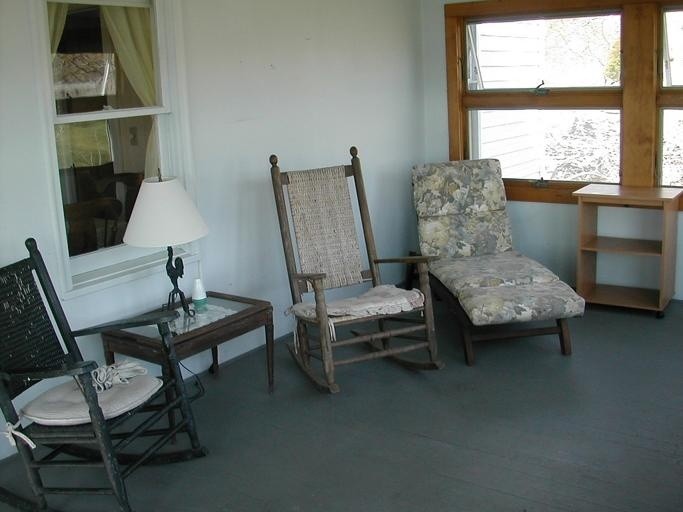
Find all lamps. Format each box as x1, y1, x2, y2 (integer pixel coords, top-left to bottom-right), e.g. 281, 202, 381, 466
121, 176, 210, 317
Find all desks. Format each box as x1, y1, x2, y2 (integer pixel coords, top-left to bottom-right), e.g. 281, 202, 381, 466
101, 290, 275, 446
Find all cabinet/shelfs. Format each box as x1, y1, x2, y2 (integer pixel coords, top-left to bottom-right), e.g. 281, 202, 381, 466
572, 183, 683, 320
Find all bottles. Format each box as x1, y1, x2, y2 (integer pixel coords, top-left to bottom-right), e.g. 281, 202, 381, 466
191, 278, 207, 305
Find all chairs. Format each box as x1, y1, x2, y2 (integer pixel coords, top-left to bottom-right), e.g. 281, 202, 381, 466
0, 237, 210, 511
269, 146, 446, 395
405, 158, 586, 366
